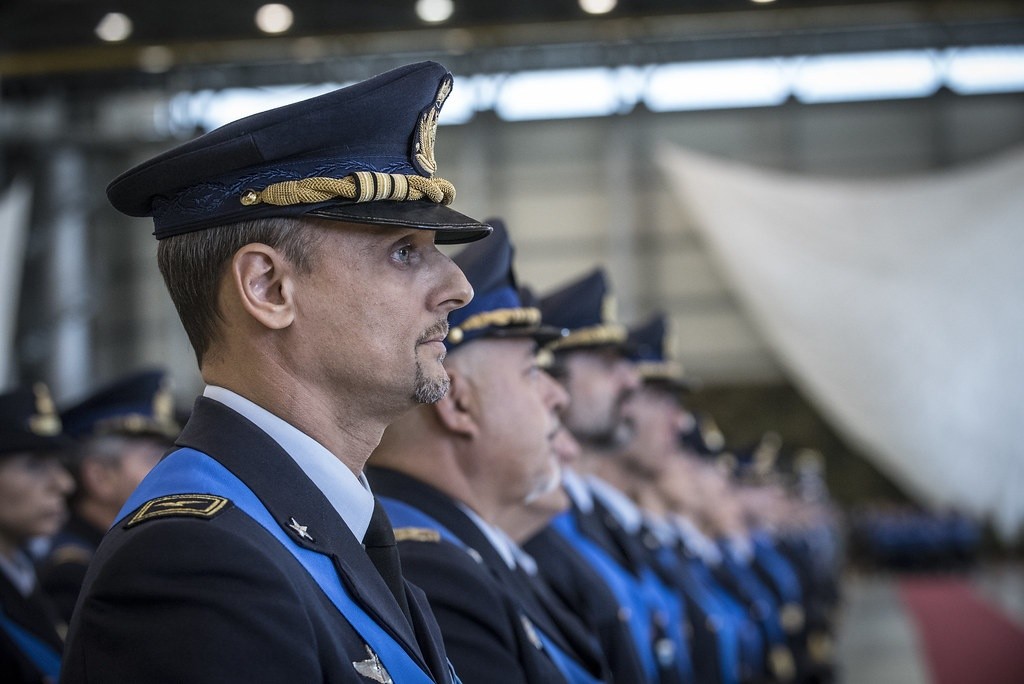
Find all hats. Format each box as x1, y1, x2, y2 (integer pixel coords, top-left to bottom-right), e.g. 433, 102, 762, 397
444, 219, 571, 351
618, 311, 688, 393
105, 61, 492, 246
60, 364, 182, 446
0, 381, 75, 454
534, 268, 648, 360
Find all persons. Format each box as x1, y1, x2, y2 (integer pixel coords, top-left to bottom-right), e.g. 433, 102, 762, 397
60, 59, 493, 684
1, 221, 975, 684
